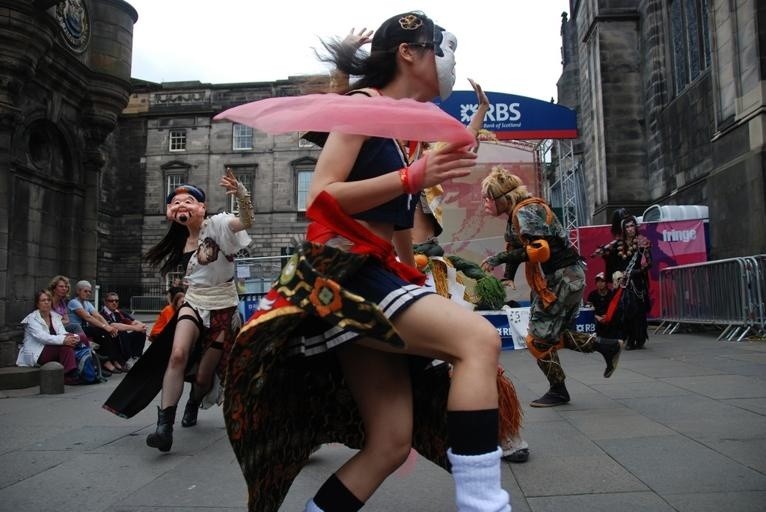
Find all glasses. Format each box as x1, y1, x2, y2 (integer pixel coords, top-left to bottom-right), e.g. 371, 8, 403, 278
107, 299, 119, 302
84, 289, 92, 292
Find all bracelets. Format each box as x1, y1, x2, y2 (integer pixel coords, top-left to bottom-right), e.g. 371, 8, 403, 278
400, 167, 412, 194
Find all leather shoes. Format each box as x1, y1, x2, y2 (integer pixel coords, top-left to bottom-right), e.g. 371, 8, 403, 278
102, 356, 141, 377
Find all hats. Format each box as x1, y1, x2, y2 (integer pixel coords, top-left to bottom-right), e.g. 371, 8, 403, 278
594, 271, 606, 280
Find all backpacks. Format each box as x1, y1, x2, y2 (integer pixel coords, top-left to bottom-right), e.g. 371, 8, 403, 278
75, 348, 107, 384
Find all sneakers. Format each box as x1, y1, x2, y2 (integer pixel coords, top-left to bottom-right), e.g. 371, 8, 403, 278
593, 336, 624, 378
625, 336, 646, 350
530, 380, 570, 407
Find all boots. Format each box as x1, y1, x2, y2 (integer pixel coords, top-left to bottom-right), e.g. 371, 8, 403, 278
181, 380, 211, 427
147, 405, 176, 452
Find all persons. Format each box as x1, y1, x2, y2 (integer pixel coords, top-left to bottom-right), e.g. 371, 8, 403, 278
214, 14, 508, 511
586, 273, 615, 338
101, 168, 256, 452
590, 208, 652, 351
325, 26, 529, 464
149, 277, 188, 343
100, 292, 147, 371
67, 280, 122, 374
17, 275, 107, 383
480, 164, 623, 407
